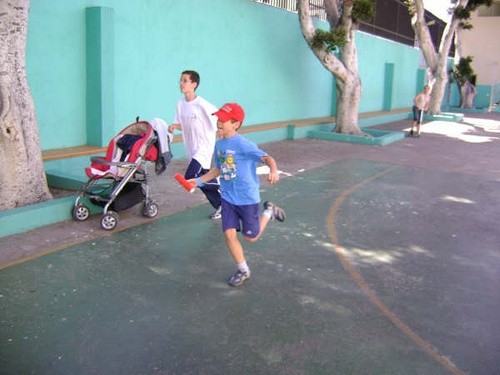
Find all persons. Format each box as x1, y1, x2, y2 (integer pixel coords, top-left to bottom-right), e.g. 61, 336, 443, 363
184, 102, 286, 288
409, 85, 431, 136
168, 69, 220, 220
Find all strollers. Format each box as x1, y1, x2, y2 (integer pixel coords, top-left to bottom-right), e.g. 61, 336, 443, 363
70, 115, 174, 231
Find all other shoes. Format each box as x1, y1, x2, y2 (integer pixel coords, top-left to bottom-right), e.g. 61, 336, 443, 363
210, 205, 223, 218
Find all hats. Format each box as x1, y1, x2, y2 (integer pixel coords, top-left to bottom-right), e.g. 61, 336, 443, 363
212, 102, 245, 122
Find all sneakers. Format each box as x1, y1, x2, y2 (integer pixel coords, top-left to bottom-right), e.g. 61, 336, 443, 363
227, 270, 252, 286
264, 199, 286, 223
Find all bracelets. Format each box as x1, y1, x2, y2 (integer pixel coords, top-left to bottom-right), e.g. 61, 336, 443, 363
196, 177, 204, 187
